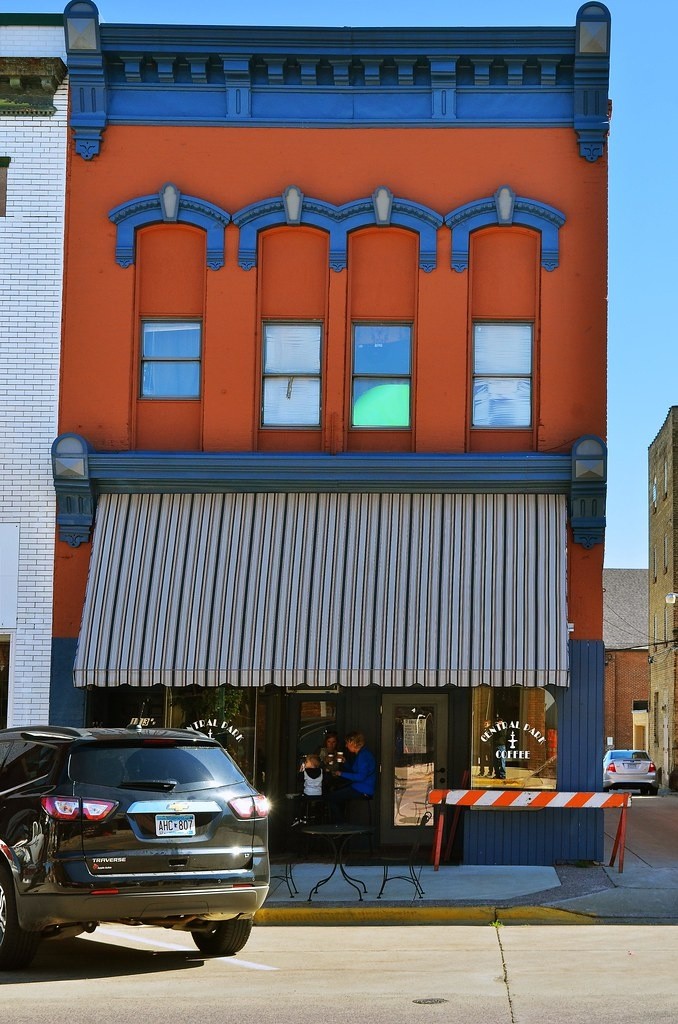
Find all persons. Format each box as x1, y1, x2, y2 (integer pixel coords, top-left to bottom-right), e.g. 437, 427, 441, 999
291, 730, 378, 828
478, 717, 509, 779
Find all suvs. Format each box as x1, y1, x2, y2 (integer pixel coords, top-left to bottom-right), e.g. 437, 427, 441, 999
0, 725, 270, 971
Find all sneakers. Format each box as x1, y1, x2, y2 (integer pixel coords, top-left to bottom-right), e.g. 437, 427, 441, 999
292, 817, 299, 826
300, 816, 307, 824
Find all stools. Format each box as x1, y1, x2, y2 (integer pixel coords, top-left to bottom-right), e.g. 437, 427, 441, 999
299, 792, 374, 863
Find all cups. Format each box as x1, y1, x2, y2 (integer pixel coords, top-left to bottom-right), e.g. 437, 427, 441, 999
337, 752, 343, 762
328, 755, 334, 765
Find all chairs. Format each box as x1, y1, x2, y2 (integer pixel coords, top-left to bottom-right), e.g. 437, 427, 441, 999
266, 841, 299, 899
377, 812, 432, 900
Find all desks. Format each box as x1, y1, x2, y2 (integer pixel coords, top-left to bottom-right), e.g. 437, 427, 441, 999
303, 822, 376, 903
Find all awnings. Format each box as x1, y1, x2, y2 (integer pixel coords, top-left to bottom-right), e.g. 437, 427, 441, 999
72, 492, 571, 688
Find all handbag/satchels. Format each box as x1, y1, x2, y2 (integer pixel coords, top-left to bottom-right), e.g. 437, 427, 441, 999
335, 779, 353, 789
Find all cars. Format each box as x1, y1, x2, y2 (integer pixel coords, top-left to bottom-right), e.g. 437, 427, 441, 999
603, 749, 660, 795
